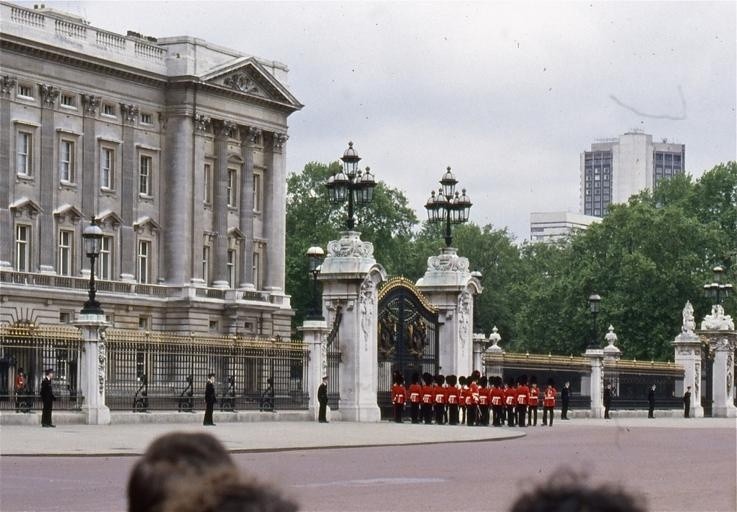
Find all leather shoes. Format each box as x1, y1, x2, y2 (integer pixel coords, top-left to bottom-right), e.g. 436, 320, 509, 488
393, 418, 553, 427
41, 424, 50, 427
49, 424, 55, 427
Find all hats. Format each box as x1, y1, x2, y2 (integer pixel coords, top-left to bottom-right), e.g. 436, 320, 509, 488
322, 376, 328, 380
45, 369, 53, 374
394, 372, 555, 387
208, 374, 215, 378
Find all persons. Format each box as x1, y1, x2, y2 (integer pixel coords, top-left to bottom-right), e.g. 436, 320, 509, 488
511, 466, 648, 512
128, 433, 232, 512
154, 472, 297, 512
15, 368, 26, 413
603, 383, 611, 419
683, 386, 691, 418
561, 381, 570, 420
318, 377, 329, 423
40, 369, 56, 427
647, 384, 656, 418
203, 374, 218, 425
391, 370, 556, 427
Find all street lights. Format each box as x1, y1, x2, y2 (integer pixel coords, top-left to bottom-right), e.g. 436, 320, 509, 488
424, 164, 474, 249
303, 246, 325, 320
702, 265, 735, 307
470, 268, 484, 332
588, 287, 602, 350
80, 214, 108, 315
322, 142, 377, 231
